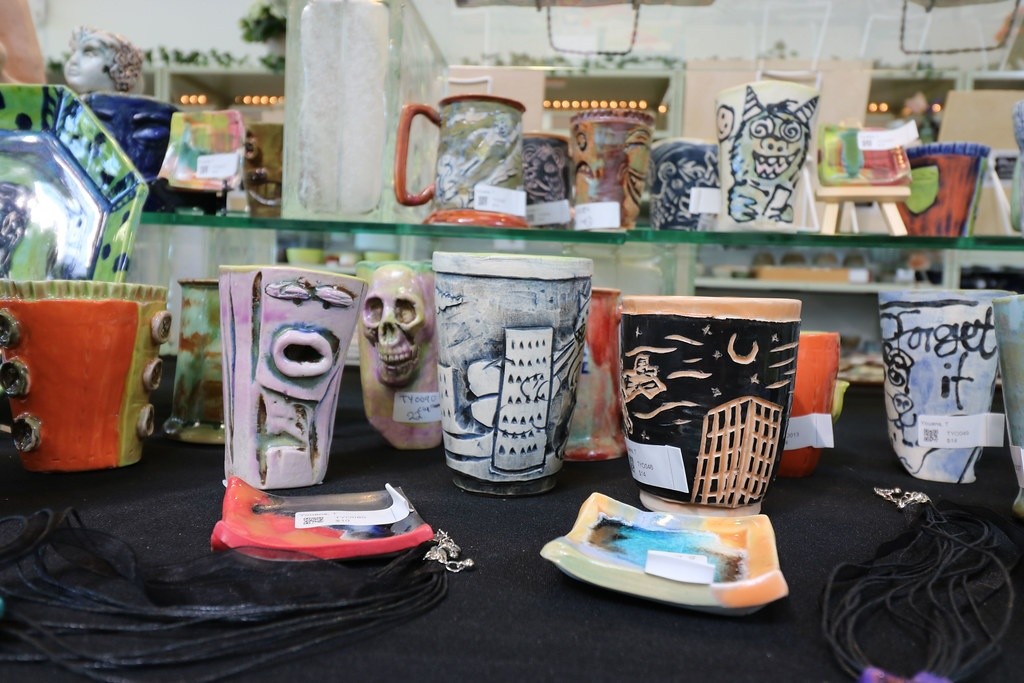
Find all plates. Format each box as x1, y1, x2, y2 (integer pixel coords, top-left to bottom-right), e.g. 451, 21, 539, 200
0, 84, 149, 284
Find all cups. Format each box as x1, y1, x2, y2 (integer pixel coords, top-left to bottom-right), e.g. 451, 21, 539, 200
218, 250, 1023, 514
0, 277, 171, 471
395, 81, 991, 237
164, 280, 224, 444
